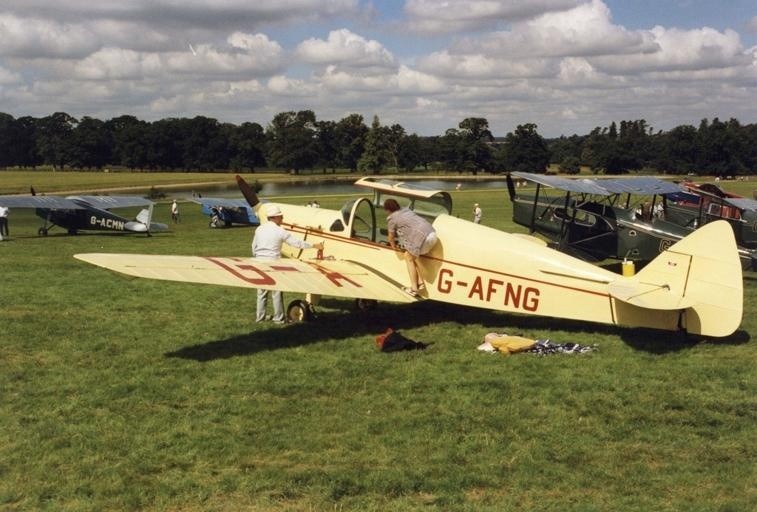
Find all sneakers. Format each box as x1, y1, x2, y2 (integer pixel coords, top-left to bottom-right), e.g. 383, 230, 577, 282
401, 286, 418, 297
257, 314, 272, 324
417, 283, 424, 290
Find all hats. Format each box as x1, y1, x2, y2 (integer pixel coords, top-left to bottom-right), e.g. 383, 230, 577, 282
267, 205, 284, 218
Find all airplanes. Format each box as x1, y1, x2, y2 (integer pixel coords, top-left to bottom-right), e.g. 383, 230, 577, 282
69, 176, 745, 337
188, 195, 271, 229
0, 186, 169, 238
505, 169, 757, 273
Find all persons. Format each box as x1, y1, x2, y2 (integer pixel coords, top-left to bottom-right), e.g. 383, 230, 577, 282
0, 206, 11, 240
172, 199, 180, 224
384, 199, 437, 296
252, 205, 324, 325
473, 203, 481, 224
208, 206, 232, 228
620, 200, 664, 221
